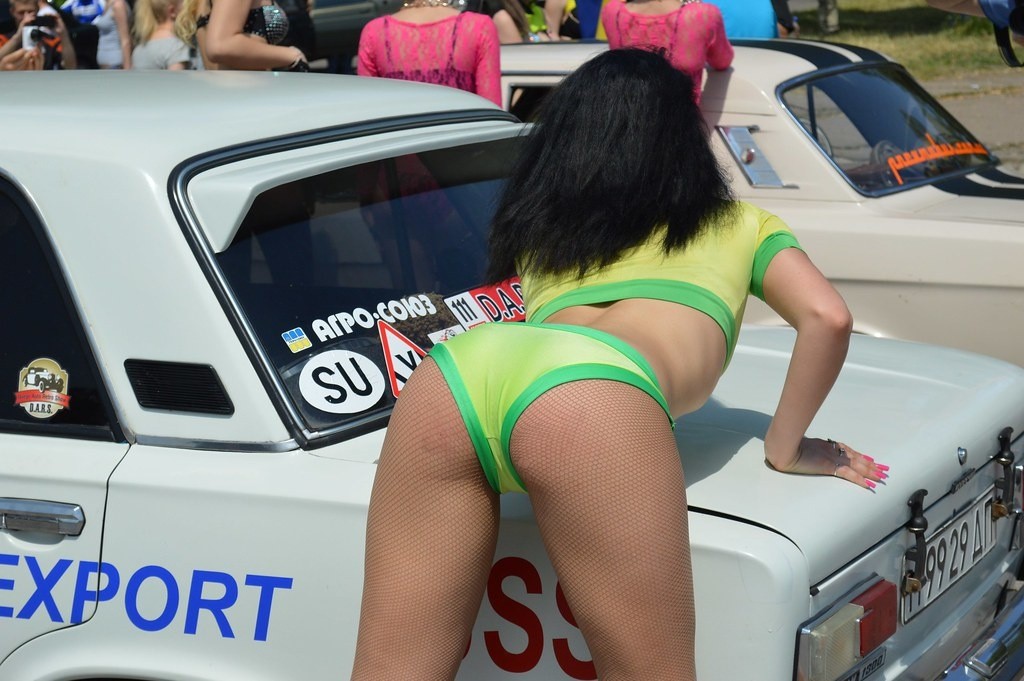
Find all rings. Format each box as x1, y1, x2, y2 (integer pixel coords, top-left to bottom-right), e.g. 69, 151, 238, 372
834, 463, 839, 476
832, 441, 835, 448
838, 448, 844, 456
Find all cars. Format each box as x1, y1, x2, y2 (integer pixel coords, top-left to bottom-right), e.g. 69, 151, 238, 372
0, 69, 1024, 681
492, 32, 1024, 374
308, 0, 406, 58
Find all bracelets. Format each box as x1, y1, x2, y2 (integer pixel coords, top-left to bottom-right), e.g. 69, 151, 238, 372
289, 46, 302, 68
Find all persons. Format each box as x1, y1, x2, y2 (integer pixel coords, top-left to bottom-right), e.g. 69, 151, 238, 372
350, 42, 888, 681
467, 0, 608, 41
601, 0, 735, 104
0, 0, 308, 73
356, 0, 503, 109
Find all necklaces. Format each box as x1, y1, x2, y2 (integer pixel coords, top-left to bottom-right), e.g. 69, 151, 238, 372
401, 0, 448, 8
